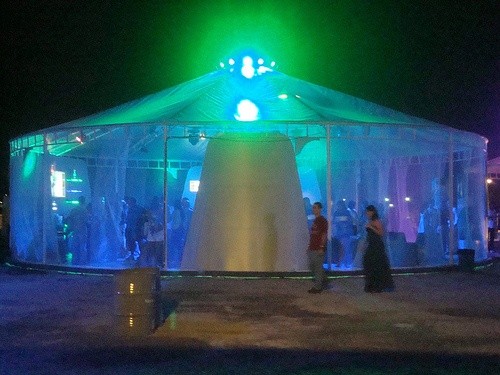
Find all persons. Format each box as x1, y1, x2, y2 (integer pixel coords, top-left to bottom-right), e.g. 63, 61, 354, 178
120, 196, 193, 269
419, 203, 459, 255
303, 196, 315, 228
67, 196, 91, 265
307, 202, 328, 293
363, 205, 396, 293
332, 198, 360, 267
488, 201, 498, 253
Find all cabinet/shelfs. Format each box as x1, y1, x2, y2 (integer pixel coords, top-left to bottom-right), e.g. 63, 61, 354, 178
65, 179, 82, 203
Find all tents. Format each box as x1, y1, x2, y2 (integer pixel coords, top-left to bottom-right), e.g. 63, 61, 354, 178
6, 63, 494, 280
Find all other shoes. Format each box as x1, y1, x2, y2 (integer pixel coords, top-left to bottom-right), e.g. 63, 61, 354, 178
323, 285, 333, 289
308, 287, 321, 292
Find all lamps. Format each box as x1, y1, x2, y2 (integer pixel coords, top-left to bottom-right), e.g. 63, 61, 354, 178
188, 128, 200, 146
